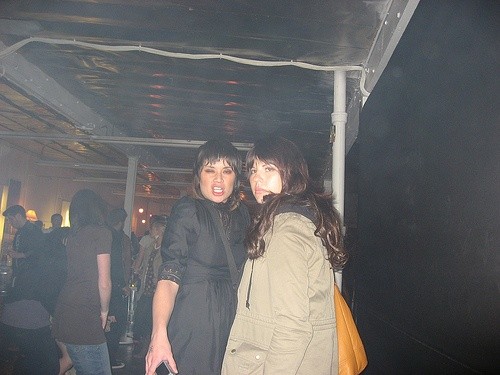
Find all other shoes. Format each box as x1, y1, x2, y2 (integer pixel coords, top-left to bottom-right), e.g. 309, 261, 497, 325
112, 361, 125, 369
134, 350, 146, 358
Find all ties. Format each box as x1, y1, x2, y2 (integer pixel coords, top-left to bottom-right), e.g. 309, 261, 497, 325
15, 234, 21, 267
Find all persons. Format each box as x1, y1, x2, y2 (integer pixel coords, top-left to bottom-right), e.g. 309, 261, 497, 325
136, 216, 164, 341
44, 213, 66, 271
3, 204, 44, 288
144, 134, 252, 375
105, 208, 131, 370
133, 214, 158, 304
51, 189, 112, 375
0, 227, 68, 375
220, 134, 349, 375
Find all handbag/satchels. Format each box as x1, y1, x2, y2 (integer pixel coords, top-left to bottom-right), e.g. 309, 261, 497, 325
334, 278, 368, 375
